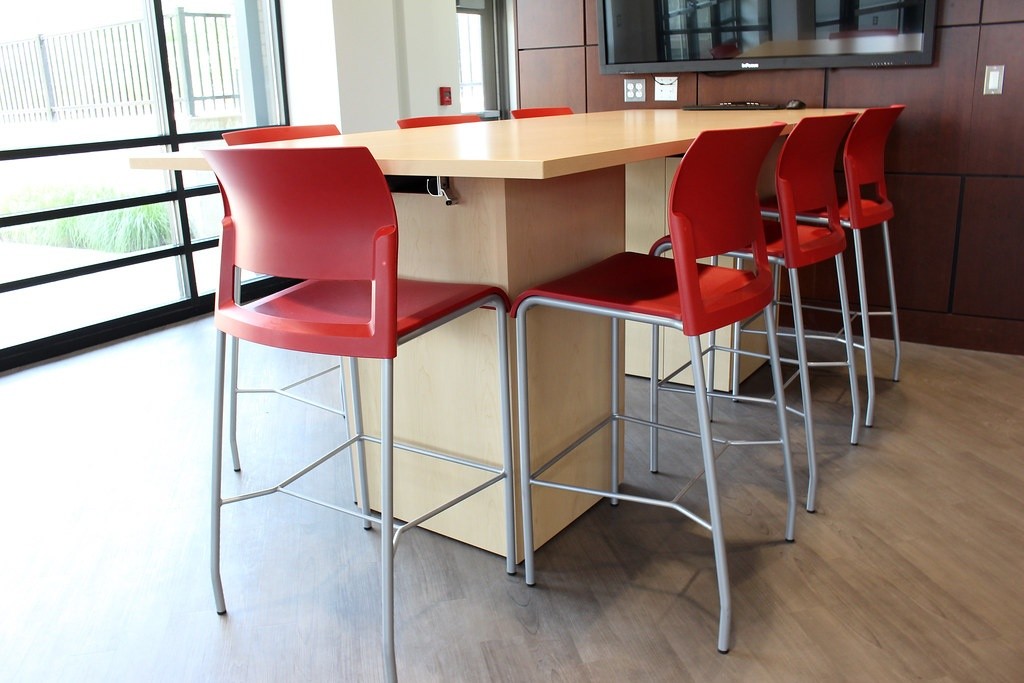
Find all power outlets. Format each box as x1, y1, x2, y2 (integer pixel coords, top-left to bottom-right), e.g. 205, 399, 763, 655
624, 79, 646, 102
655, 77, 678, 101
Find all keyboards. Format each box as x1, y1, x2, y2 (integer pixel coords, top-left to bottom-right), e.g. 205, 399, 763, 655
683, 101, 779, 110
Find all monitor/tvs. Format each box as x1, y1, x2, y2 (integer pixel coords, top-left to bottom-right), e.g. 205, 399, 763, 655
596, 0, 938, 75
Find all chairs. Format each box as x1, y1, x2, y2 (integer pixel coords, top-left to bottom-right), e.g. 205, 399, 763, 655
222, 107, 574, 472
830, 29, 898, 39
197, 146, 515, 683
509, 103, 906, 653
710, 43, 741, 58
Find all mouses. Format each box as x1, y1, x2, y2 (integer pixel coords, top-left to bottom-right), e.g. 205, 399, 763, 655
786, 99, 805, 109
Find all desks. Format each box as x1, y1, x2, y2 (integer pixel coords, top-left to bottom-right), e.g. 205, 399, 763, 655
130, 108, 867, 566
735, 33, 923, 58
